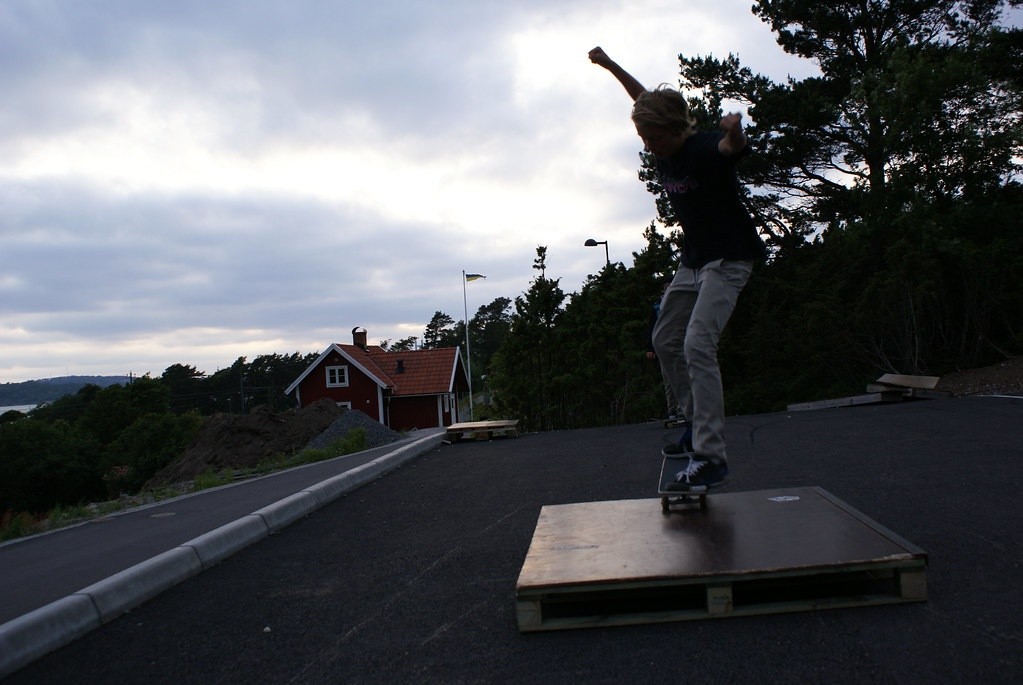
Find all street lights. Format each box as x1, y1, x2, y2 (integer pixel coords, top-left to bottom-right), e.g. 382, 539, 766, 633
585, 239, 610, 266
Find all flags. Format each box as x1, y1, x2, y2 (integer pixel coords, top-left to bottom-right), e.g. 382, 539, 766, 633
466, 274, 486, 282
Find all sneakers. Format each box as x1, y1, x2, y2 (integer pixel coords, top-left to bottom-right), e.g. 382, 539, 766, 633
662, 424, 694, 458
674, 456, 733, 491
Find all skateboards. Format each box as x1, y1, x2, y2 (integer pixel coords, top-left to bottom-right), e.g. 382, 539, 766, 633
658, 452, 710, 512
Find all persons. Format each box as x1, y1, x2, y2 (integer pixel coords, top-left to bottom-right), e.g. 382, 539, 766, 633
589, 46, 766, 493
